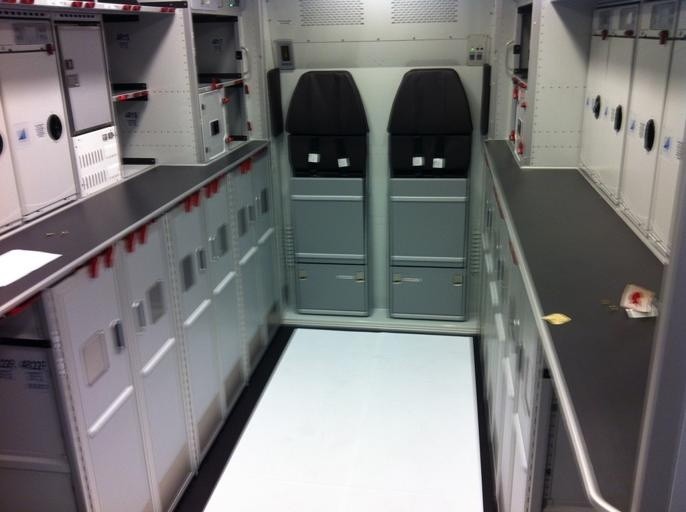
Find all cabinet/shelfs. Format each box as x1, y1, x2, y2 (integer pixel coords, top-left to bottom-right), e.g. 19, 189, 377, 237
1, 0, 286, 511
482, 2, 685, 511
